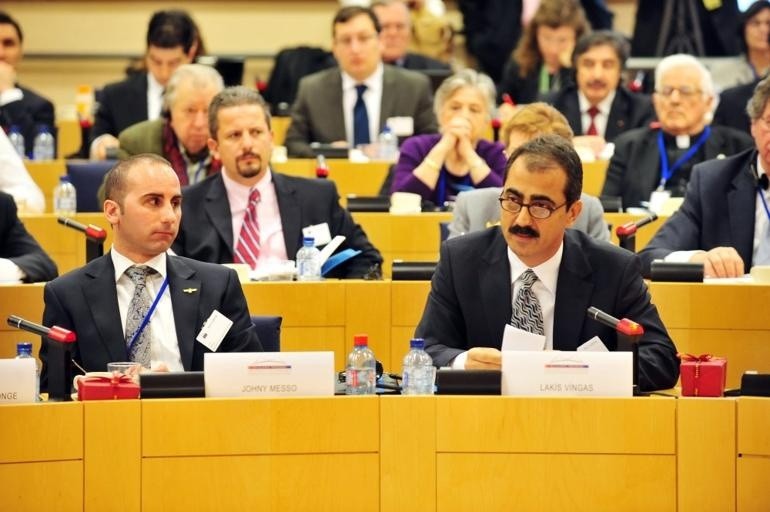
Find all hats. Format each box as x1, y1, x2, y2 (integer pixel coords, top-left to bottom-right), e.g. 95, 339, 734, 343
122, 265, 157, 370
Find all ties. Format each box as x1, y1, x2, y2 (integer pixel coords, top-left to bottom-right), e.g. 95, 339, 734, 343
586, 105, 601, 134
353, 83, 372, 148
230, 187, 262, 270
511, 267, 545, 334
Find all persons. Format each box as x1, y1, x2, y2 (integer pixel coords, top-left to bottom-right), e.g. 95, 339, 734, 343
0, 11, 57, 160
38, 152, 264, 398
170, 85, 382, 279
414, 134, 680, 392
118, 64, 226, 189
84, 9, 201, 161
0, 126, 47, 215
0, 190, 59, 284
284, 6, 439, 162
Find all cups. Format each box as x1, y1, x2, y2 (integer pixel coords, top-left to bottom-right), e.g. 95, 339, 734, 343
106, 361, 141, 398
390, 192, 421, 214
73, 371, 112, 393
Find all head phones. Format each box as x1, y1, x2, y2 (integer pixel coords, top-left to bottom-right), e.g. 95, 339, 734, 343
747, 160, 769, 189
160, 105, 171, 119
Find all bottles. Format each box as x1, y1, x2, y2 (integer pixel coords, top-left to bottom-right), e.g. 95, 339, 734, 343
344, 333, 377, 396
11, 341, 41, 402
53, 175, 78, 214
377, 127, 400, 160
294, 237, 321, 279
6, 125, 25, 160
315, 155, 329, 179
401, 338, 435, 395
32, 125, 56, 160
76, 83, 96, 126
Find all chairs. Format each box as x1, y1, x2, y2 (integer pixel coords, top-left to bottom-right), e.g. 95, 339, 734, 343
250, 315, 284, 354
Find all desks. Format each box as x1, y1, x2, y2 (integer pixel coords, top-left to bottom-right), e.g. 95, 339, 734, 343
56, 120, 83, 159
13, 160, 767, 277
269, 116, 292, 146
0, 278, 770, 391
0, 394, 769, 511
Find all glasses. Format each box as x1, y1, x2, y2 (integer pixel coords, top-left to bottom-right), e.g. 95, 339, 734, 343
499, 190, 569, 220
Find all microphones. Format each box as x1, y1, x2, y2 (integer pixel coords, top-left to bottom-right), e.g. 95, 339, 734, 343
314, 154, 330, 178
629, 70, 646, 92
587, 306, 644, 395
7, 315, 78, 398
77, 112, 91, 158
255, 74, 268, 95
57, 217, 107, 264
616, 213, 658, 252
491, 110, 501, 141
